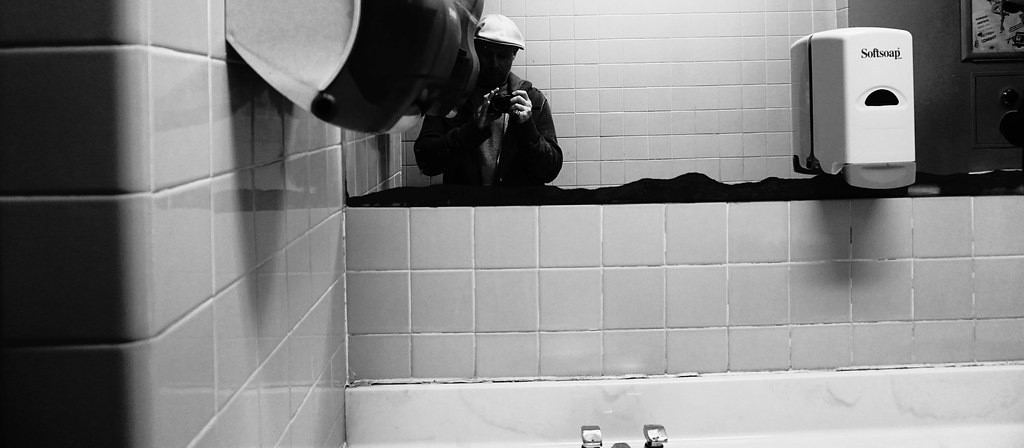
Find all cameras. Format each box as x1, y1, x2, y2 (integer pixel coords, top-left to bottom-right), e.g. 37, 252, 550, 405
487, 93, 512, 120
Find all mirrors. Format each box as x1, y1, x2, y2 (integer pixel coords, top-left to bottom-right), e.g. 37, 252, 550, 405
344, 0, 1024, 198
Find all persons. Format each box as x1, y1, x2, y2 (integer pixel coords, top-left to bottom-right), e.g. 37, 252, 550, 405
413, 15, 563, 206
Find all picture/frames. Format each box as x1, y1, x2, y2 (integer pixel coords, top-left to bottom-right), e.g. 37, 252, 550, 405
959, 0, 1024, 63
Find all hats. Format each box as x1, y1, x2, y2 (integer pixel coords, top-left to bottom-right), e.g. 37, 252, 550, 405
473, 14, 525, 50
